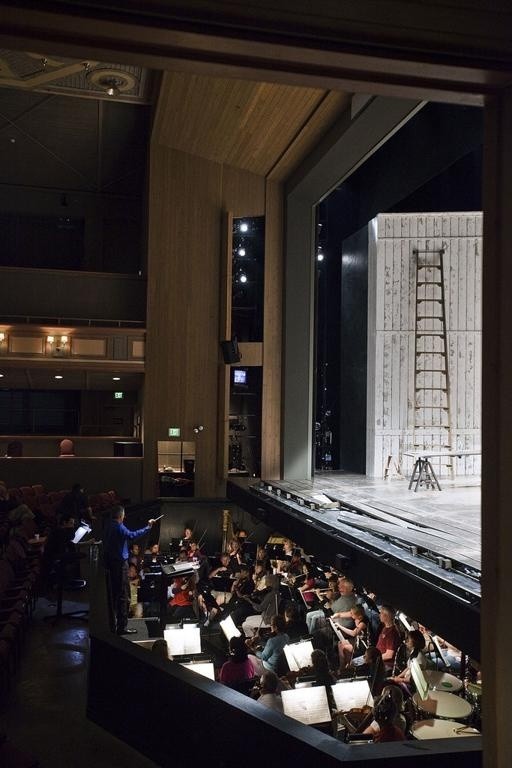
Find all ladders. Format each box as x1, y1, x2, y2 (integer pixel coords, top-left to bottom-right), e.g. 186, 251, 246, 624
413, 250, 455, 487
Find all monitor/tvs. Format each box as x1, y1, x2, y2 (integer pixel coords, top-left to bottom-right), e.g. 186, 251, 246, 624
234, 370, 247, 384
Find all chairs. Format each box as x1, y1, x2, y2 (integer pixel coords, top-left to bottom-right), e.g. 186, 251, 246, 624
0, 734, 40, 767
6, 439, 22, 456
57, 438, 78, 457
0, 479, 120, 710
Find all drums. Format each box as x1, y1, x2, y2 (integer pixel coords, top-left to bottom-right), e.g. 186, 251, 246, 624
411, 689, 473, 720
410, 718, 481, 741
422, 668, 463, 691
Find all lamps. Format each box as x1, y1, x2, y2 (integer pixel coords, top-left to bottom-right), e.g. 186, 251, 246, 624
46, 335, 68, 355
230, 216, 256, 303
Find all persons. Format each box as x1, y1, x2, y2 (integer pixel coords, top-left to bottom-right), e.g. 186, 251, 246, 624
103, 504, 156, 635
44, 515, 96, 590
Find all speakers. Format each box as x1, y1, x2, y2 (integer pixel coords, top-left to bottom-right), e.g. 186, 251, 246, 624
114, 441, 142, 457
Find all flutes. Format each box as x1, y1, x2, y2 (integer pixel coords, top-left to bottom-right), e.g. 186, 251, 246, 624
282, 572, 309, 583
303, 587, 335, 594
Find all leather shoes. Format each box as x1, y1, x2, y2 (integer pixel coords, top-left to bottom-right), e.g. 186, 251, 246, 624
117, 628, 138, 635
